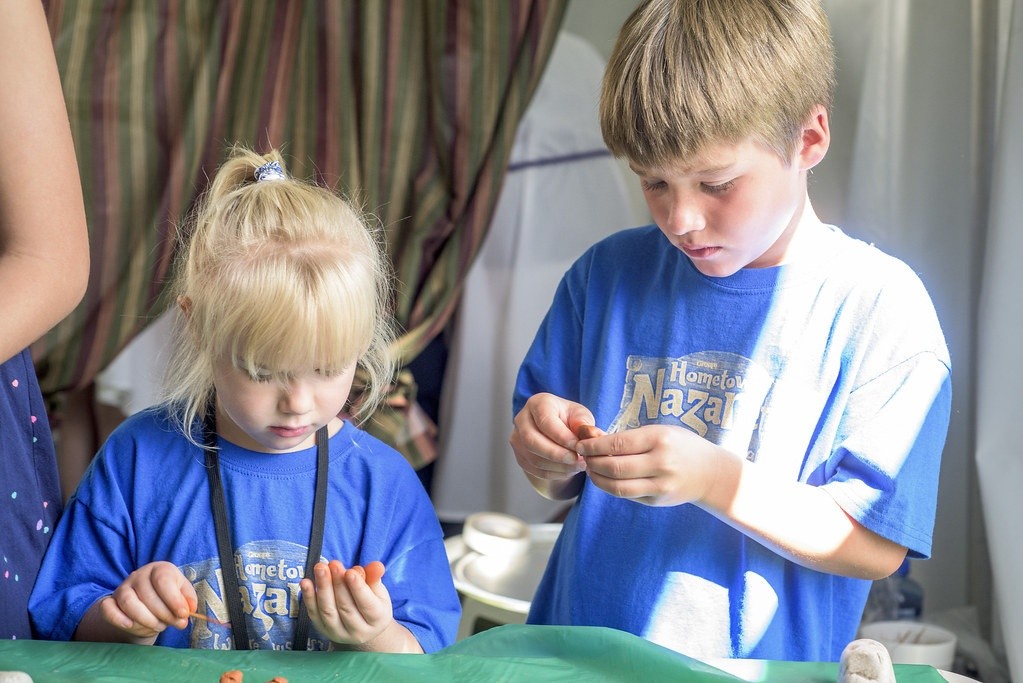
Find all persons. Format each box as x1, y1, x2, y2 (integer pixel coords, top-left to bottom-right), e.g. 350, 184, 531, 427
0, 0, 91, 641
27, 141, 462, 656
506, 0, 953, 663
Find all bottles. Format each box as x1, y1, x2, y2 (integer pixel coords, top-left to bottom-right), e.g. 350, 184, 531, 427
894, 559, 923, 621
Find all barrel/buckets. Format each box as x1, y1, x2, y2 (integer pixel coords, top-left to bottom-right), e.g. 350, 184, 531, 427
864, 620, 958, 673
